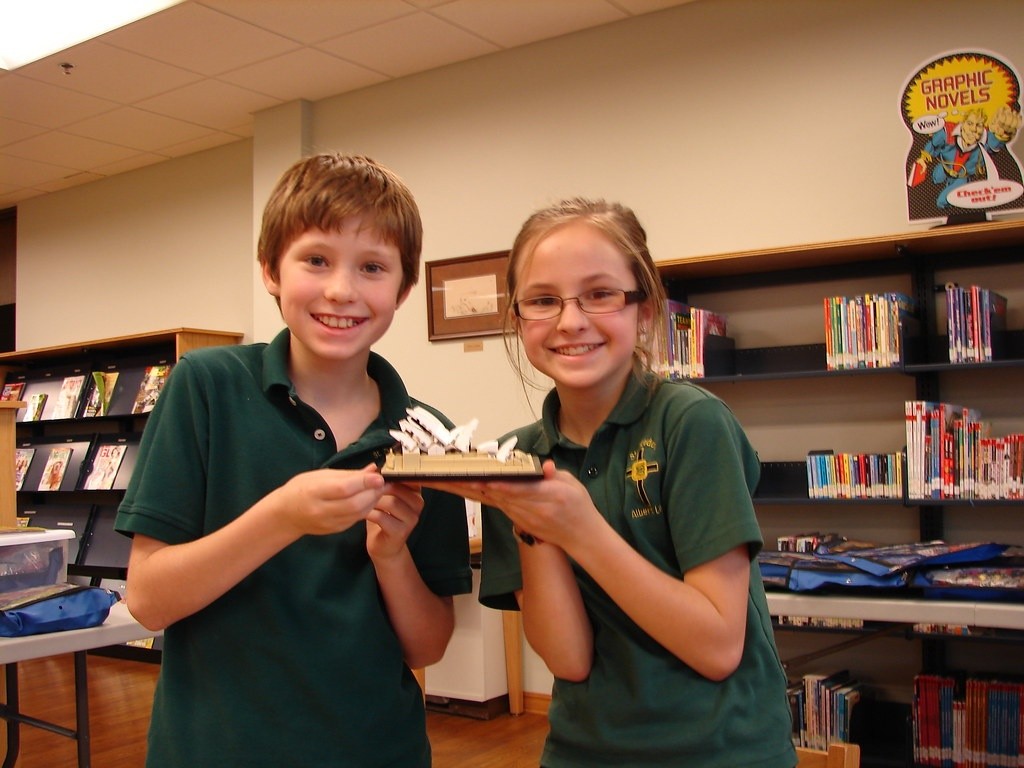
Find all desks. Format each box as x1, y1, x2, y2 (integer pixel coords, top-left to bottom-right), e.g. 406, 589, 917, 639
0, 606, 161, 767
399, 536, 525, 721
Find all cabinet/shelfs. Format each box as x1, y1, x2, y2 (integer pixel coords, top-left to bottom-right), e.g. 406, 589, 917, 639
0, 327, 246, 665
651, 218, 1024, 768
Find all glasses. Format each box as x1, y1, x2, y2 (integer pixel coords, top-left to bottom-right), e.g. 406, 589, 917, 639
513, 286, 647, 321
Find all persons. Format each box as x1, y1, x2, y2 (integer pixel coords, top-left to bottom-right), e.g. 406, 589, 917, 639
112, 151, 476, 767
477, 193, 799, 768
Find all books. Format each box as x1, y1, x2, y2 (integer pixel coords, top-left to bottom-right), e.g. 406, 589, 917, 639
23, 394, 48, 422
806, 452, 904, 500
38, 448, 72, 491
16, 448, 35, 491
83, 445, 127, 490
2, 383, 26, 401
910, 674, 1024, 768
51, 376, 85, 418
797, 674, 864, 751
131, 366, 170, 414
944, 286, 1008, 362
80, 371, 119, 416
822, 291, 914, 369
638, 298, 726, 379
904, 401, 1024, 500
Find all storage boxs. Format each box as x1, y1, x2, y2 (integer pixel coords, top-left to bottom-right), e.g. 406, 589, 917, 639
0, 528, 74, 598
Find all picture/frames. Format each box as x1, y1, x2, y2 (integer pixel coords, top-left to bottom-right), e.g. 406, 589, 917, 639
424, 247, 512, 342
